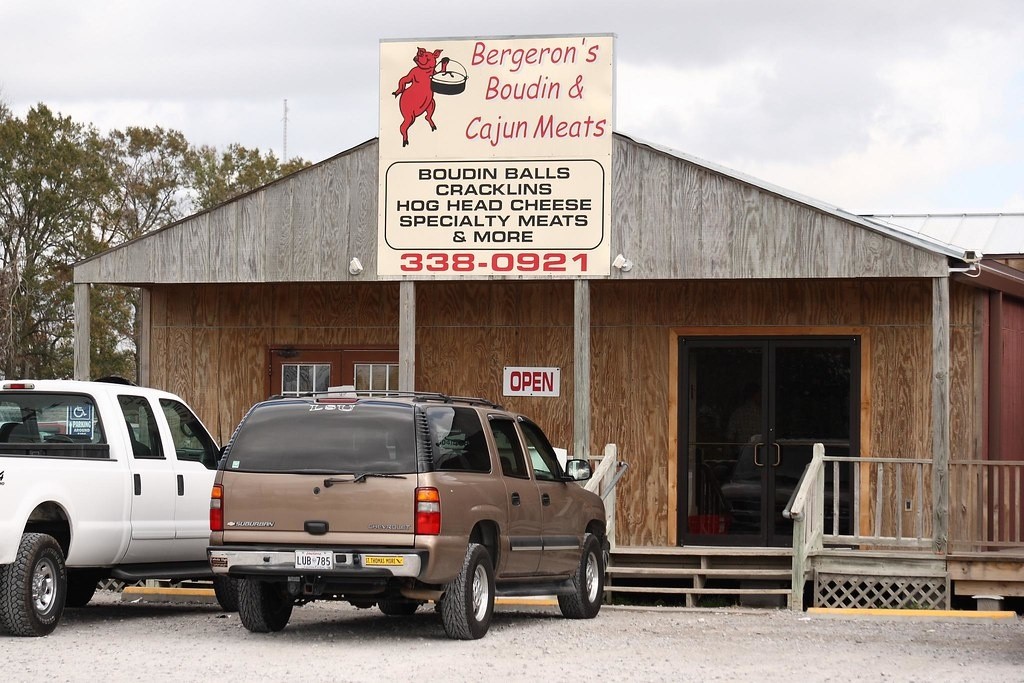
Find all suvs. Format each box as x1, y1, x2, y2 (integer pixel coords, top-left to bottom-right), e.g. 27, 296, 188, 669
698, 434, 850, 532
0, 375, 228, 638
206, 388, 611, 641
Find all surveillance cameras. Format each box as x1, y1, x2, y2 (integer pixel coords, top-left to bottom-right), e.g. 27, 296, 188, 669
964, 248, 984, 262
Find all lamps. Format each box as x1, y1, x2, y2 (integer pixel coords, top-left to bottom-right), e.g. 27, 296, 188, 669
612, 253, 632, 271
349, 257, 364, 275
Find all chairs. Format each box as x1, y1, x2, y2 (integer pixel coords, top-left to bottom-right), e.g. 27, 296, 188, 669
0, 423, 32, 443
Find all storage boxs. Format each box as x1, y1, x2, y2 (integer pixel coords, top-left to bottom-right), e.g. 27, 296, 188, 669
688, 514, 732, 535
